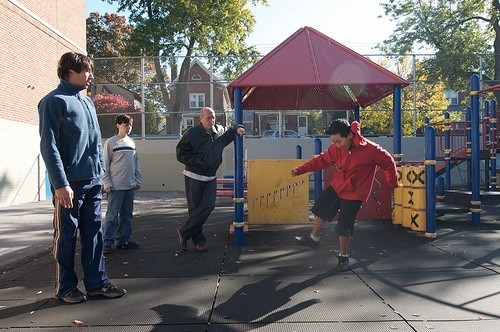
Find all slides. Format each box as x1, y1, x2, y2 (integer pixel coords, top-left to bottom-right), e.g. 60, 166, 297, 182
435, 148, 466, 178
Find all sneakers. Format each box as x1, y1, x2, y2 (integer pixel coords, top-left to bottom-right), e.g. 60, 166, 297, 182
87, 283, 127, 299
295, 234, 321, 249
56, 288, 87, 304
335, 256, 350, 272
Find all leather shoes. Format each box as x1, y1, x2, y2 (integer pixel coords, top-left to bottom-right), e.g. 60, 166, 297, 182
176, 228, 187, 252
195, 242, 208, 251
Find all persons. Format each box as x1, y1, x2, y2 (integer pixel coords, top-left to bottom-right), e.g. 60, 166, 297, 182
290, 118, 402, 272
175, 106, 246, 252
101, 114, 141, 254
37, 53, 126, 302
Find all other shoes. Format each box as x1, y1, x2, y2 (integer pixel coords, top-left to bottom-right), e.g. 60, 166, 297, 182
103, 245, 114, 254
115, 241, 140, 249
